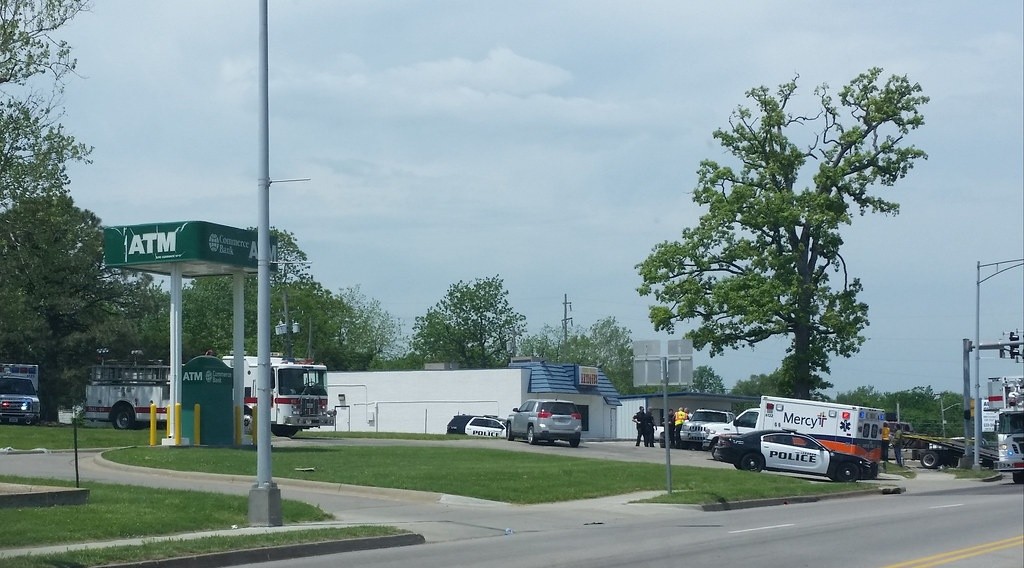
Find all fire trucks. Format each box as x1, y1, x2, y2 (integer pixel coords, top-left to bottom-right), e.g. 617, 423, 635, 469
84, 349, 337, 437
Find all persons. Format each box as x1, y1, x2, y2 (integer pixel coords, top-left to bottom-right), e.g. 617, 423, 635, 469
892, 424, 905, 464
668, 409, 676, 448
674, 408, 688, 449
684, 408, 692, 421
882, 422, 890, 461
632, 406, 657, 447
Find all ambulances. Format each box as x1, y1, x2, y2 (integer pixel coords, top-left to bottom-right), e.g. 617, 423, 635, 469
715, 395, 885, 462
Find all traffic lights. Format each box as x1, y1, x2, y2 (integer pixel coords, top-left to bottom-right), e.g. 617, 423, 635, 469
1009, 332, 1019, 359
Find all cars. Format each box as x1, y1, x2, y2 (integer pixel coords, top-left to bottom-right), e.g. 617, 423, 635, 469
713, 428, 878, 482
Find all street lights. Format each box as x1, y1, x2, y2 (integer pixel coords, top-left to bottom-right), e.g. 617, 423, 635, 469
941, 403, 960, 437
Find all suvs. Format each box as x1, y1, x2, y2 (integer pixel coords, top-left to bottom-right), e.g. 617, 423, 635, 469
506, 399, 582, 446
446, 414, 507, 438
680, 408, 736, 450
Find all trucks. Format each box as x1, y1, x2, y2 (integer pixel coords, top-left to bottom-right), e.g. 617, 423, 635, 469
0, 363, 40, 424
995, 409, 1024, 483
884, 421, 999, 470
